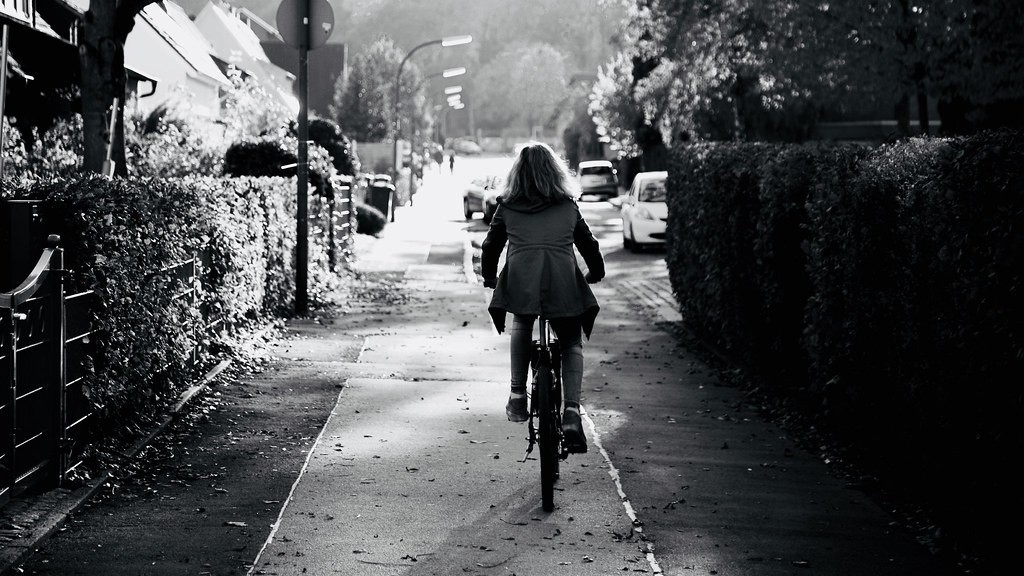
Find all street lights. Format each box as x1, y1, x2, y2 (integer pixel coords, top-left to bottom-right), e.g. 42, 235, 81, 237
410, 67, 465, 205
392, 36, 472, 220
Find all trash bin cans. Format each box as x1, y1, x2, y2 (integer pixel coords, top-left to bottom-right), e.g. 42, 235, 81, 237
372, 182, 396, 222
374, 174, 394, 222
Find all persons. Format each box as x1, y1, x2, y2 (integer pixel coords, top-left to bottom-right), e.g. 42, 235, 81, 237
481, 145, 606, 454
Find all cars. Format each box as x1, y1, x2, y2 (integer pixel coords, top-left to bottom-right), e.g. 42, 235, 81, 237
462, 166, 512, 222
618, 169, 669, 252
573, 161, 620, 201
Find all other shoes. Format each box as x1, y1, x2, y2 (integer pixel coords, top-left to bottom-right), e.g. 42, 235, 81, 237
562, 410, 588, 454
505, 392, 529, 422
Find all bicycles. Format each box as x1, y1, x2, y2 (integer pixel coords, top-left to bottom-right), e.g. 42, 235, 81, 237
486, 279, 598, 514
